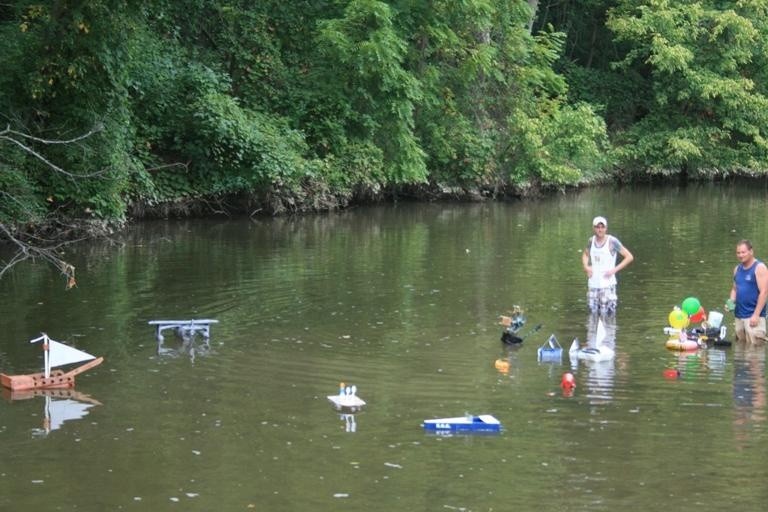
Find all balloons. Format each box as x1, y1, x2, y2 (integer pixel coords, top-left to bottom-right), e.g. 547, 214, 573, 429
668, 296, 706, 329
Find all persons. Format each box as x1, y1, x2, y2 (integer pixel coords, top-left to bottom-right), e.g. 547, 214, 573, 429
581, 215, 635, 315
724, 239, 768, 347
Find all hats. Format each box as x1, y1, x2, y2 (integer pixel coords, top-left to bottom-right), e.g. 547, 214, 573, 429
592, 216, 607, 228
